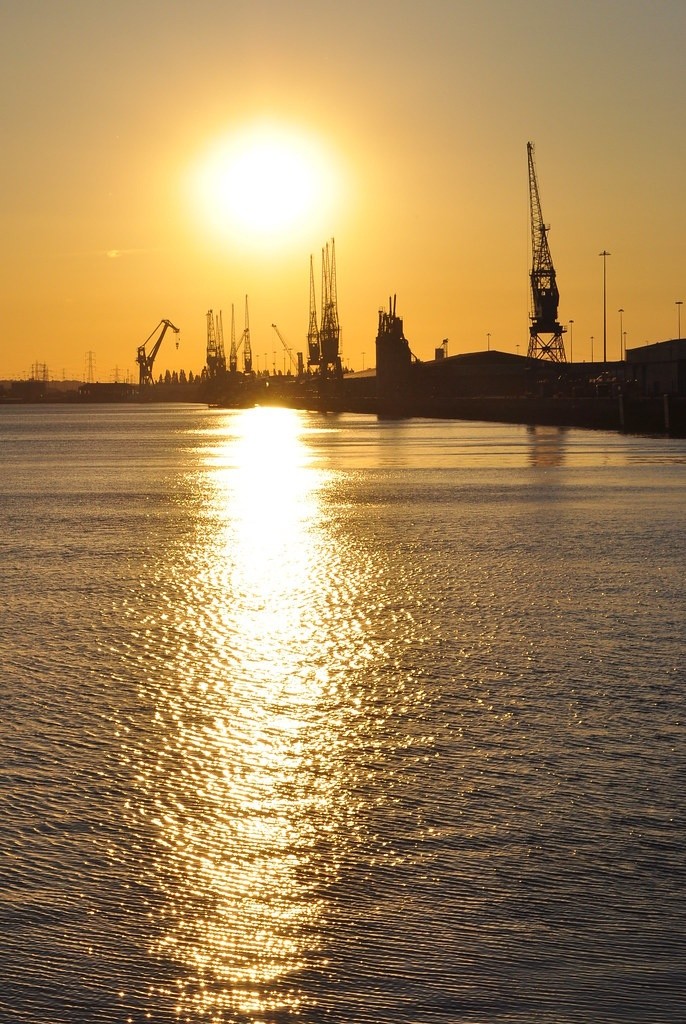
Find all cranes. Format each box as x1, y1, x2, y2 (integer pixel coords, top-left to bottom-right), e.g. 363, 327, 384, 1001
205, 292, 254, 378
524, 138, 570, 370
272, 324, 299, 369
135, 317, 180, 401
304, 235, 349, 383
